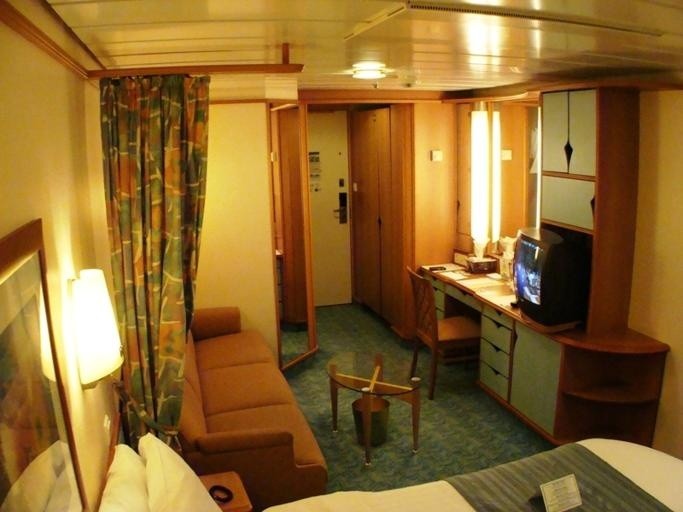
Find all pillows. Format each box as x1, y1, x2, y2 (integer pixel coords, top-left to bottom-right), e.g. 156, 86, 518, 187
97, 432, 223, 512
0, 440, 82, 511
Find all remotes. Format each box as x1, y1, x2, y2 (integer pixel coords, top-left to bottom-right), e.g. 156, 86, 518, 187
511, 301, 519, 308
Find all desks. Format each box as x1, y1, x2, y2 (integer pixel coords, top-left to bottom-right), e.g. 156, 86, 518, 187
422, 263, 670, 447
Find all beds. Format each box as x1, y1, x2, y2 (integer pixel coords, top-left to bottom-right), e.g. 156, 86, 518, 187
95, 411, 683, 512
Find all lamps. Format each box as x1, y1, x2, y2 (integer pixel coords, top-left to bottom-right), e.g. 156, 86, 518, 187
67, 268, 125, 390
470, 102, 491, 259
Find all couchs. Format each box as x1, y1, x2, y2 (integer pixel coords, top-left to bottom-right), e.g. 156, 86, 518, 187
179, 306, 328, 508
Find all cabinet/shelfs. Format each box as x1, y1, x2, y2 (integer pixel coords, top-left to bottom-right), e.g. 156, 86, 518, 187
347, 103, 416, 340
540, 174, 596, 231
540, 88, 597, 176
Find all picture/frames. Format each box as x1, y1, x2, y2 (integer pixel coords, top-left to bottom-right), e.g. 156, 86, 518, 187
0, 218, 89, 512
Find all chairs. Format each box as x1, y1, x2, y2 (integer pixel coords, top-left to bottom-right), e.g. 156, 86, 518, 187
405, 266, 480, 400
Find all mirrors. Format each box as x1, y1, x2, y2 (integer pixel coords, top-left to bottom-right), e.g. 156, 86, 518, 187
492, 101, 541, 256
268, 103, 316, 370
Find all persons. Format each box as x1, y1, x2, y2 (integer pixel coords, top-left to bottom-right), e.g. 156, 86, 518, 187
512, 243, 540, 306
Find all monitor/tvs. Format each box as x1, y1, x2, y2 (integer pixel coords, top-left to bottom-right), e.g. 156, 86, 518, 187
512, 226, 592, 326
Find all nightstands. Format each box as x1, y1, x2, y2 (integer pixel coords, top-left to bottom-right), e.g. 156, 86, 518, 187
198, 472, 252, 512
